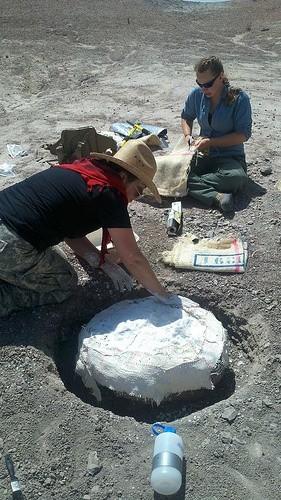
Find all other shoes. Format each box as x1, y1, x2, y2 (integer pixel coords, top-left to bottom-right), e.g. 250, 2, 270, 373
214, 193, 234, 213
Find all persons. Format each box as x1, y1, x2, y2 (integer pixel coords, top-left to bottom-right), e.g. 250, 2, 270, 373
181, 56, 253, 212
0, 138, 201, 319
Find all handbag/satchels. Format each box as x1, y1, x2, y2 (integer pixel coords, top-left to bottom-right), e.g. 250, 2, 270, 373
50, 126, 117, 165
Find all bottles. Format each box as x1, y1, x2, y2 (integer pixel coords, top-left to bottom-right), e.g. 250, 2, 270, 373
150, 423, 183, 496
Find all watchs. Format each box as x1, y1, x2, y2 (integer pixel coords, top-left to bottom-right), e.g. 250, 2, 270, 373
185, 133, 191, 137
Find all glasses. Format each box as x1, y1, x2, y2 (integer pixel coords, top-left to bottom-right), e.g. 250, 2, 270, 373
196, 73, 220, 88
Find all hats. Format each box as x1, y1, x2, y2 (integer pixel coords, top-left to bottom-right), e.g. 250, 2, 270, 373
90, 138, 163, 203
